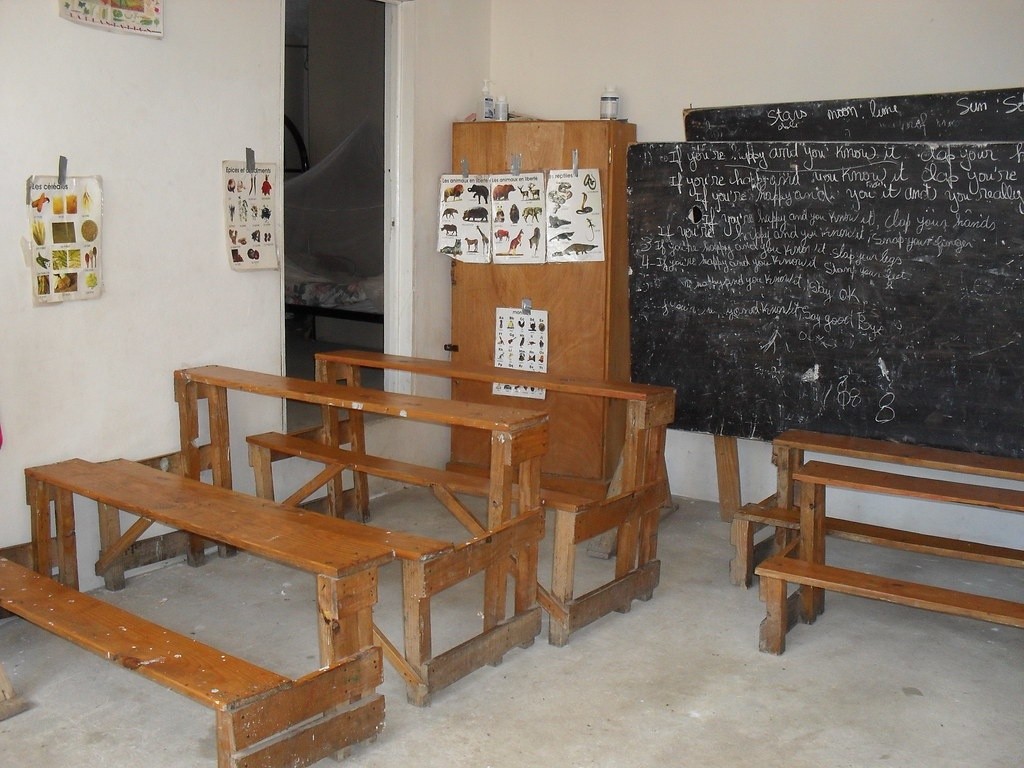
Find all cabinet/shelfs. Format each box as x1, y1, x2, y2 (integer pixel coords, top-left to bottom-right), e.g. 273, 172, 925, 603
444, 117, 639, 514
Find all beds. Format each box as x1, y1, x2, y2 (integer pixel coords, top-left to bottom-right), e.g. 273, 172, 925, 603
284, 302, 384, 325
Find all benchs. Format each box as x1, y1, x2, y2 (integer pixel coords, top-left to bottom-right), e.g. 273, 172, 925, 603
0, 350, 1023, 768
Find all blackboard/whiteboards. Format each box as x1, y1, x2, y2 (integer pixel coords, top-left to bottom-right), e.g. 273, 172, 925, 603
624, 142, 1024, 464
681, 86, 1024, 144
